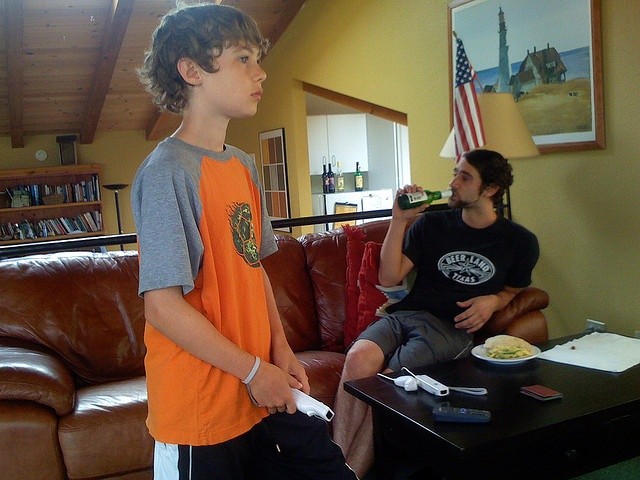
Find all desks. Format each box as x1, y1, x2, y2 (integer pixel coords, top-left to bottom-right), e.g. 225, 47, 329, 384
0, 231, 137, 250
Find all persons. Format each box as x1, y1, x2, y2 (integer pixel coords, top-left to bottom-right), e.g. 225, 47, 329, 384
332, 148, 540, 479
130, 3, 362, 480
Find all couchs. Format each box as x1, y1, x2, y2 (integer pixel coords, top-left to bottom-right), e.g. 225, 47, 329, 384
0, 204, 547, 480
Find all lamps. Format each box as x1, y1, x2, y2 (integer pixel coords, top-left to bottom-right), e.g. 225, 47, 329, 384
438, 92, 541, 219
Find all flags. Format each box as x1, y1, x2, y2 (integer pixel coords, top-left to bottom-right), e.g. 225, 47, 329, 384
453, 38, 487, 164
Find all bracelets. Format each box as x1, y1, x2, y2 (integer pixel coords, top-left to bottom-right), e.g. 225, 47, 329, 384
241, 356, 261, 385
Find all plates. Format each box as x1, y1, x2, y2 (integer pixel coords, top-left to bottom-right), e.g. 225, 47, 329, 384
471, 344, 541, 365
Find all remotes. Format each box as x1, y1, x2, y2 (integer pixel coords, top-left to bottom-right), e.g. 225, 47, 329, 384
432, 406, 491, 422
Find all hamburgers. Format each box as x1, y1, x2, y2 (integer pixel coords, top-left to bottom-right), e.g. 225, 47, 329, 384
482, 334, 537, 360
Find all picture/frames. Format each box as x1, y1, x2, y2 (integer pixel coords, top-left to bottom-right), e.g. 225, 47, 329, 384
446, 1, 607, 154
256, 127, 291, 233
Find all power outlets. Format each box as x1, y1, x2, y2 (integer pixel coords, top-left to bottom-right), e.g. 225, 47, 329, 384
586, 319, 605, 334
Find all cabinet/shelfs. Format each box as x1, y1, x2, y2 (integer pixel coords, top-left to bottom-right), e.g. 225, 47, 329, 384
369, 118, 410, 201
306, 112, 369, 176
311, 187, 395, 232
0, 163, 108, 255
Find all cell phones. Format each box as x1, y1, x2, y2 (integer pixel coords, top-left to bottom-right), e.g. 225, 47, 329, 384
520, 384, 562, 401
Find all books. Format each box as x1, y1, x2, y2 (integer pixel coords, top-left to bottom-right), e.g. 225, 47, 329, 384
0, 211, 102, 241
5, 174, 101, 205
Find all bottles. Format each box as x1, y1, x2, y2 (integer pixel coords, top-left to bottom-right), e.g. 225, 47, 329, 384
327, 163, 335, 193
336, 161, 345, 191
354, 162, 364, 191
397, 189, 453, 210
322, 164, 329, 193
